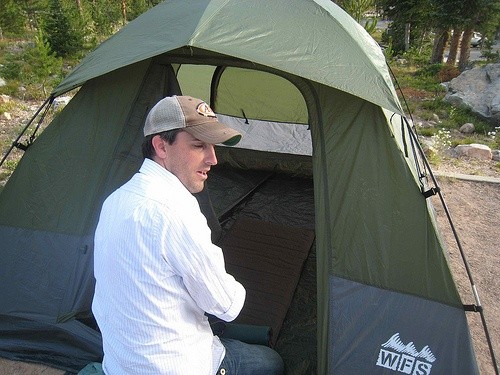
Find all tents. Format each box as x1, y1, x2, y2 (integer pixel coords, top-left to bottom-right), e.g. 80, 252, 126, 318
0, 0, 500, 375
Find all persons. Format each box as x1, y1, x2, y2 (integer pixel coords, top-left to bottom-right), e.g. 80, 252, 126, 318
76, 94, 284, 375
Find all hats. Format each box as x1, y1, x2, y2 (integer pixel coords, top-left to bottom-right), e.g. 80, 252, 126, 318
144, 95, 242, 146
